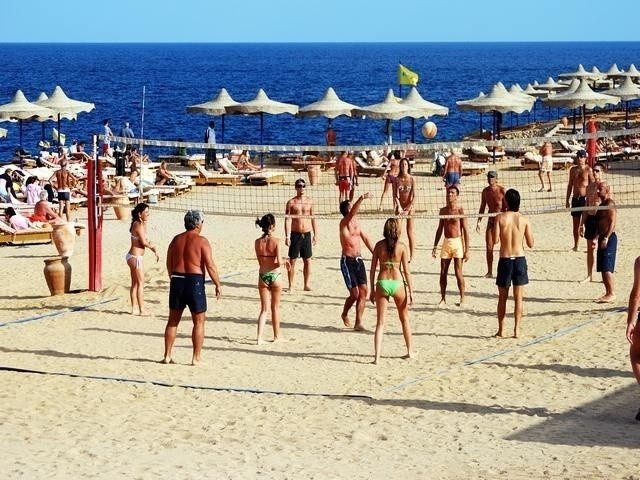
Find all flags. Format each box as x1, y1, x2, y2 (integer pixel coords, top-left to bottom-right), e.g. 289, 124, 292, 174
399, 67, 419, 85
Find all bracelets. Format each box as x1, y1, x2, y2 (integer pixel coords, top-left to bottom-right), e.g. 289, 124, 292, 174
476, 170, 507, 278
603, 236, 608, 240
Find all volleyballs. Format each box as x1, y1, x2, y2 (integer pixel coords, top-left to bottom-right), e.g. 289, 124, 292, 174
422, 122, 437, 138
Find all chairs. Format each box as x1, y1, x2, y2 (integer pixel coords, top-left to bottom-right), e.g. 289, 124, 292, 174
290, 160, 337, 171
1, 147, 284, 244
354, 112, 640, 178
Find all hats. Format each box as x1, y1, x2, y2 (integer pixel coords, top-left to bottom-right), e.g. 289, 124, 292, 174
576, 150, 586, 156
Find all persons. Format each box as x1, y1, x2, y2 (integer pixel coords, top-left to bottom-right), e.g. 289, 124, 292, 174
392, 157, 415, 263
204, 120, 220, 171
369, 217, 415, 366
565, 150, 593, 251
593, 182, 617, 303
285, 178, 318, 293
493, 189, 534, 338
580, 162, 605, 283
432, 185, 471, 307
255, 213, 282, 345
537, 141, 553, 192
325, 124, 463, 210
0, 119, 187, 231
126, 203, 159, 315
237, 150, 260, 171
339, 191, 374, 330
625, 255, 640, 424
163, 209, 222, 365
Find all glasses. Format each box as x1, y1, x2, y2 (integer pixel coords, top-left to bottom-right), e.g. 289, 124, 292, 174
487, 175, 494, 178
592, 169, 599, 172
297, 184, 306, 188
